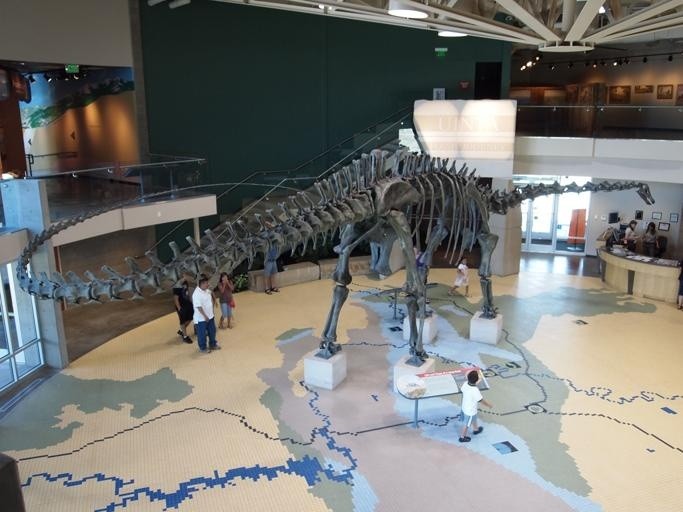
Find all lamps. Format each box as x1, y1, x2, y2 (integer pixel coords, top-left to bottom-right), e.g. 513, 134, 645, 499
388, 0, 431, 19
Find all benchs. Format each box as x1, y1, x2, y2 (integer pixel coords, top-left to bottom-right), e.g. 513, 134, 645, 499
248, 262, 319, 292
318, 256, 379, 280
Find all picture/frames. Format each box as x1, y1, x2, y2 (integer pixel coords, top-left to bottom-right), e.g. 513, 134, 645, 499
670, 213, 678, 222
609, 86, 630, 104
652, 212, 662, 219
658, 222, 670, 231
657, 85, 673, 99
675, 84, 683, 106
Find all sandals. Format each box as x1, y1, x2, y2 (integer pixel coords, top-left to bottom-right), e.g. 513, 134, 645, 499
264, 287, 280, 295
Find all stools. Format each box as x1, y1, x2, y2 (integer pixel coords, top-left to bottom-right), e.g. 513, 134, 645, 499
567, 236, 586, 244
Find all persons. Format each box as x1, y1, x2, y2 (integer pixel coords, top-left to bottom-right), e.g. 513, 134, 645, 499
263, 248, 279, 295
624, 219, 637, 252
416, 250, 428, 285
677, 259, 682, 309
191, 278, 220, 353
642, 222, 661, 257
171, 280, 192, 344
217, 272, 235, 330
447, 257, 469, 297
458, 370, 491, 442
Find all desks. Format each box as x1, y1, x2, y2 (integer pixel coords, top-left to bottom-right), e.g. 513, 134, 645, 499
396, 367, 490, 429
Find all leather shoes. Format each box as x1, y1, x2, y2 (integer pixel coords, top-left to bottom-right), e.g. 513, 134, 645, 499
459, 436, 471, 442
198, 344, 221, 353
473, 426, 483, 434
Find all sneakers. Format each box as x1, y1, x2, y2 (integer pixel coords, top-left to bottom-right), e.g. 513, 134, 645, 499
177, 330, 192, 343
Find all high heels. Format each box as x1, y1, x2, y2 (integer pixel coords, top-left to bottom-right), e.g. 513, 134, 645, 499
218, 322, 233, 329
